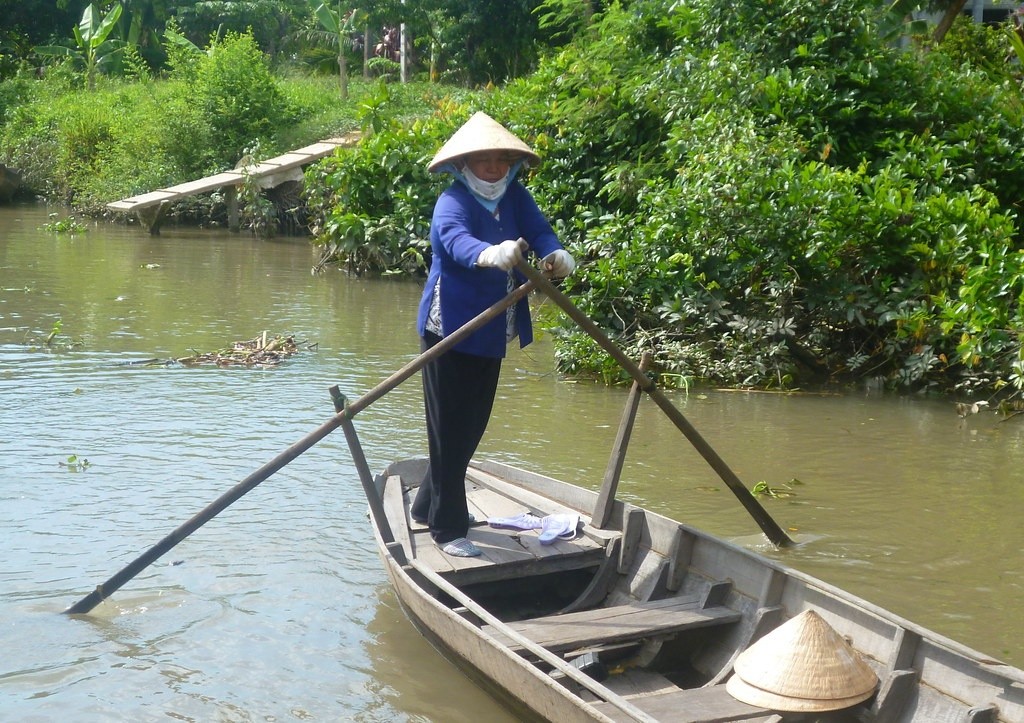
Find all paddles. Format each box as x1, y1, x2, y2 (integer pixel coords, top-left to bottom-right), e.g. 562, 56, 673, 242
60, 254, 558, 615
513, 237, 799, 550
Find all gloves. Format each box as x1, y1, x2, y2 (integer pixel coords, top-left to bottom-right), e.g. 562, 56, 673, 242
476, 240, 522, 271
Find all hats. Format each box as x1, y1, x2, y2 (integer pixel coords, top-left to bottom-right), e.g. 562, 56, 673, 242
726, 672, 875, 712
428, 111, 530, 173
733, 609, 878, 700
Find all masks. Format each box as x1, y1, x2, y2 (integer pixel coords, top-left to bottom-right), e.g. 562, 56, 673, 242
557, 515, 580, 541
461, 158, 510, 200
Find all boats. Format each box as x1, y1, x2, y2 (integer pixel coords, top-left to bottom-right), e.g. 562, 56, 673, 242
328, 350, 1024, 723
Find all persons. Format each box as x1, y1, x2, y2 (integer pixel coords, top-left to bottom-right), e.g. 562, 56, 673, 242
411, 111, 574, 557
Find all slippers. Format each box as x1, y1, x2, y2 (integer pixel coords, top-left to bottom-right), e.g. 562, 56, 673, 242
487, 512, 542, 530
538, 515, 569, 545
